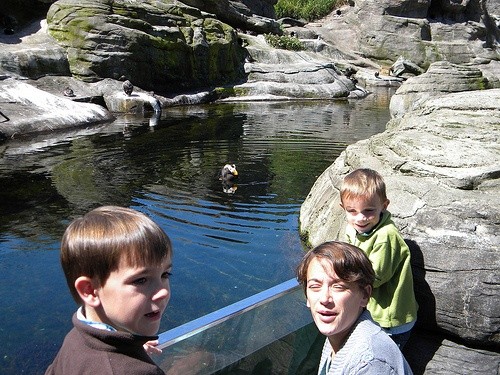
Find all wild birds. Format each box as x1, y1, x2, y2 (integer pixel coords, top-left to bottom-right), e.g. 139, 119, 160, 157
221, 164, 238, 181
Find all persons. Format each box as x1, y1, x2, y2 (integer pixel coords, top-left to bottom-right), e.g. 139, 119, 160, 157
293, 239, 413, 375
338, 167, 418, 354
43, 204, 174, 375
